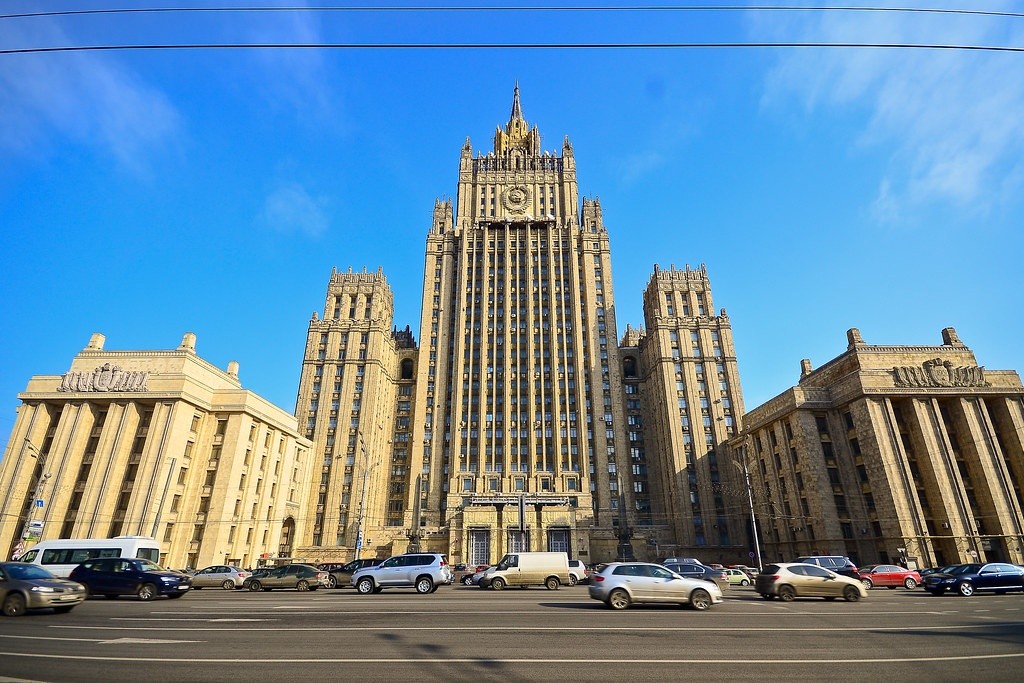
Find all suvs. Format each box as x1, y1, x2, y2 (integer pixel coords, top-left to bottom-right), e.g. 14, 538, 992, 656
351, 552, 452, 593
793, 555, 860, 579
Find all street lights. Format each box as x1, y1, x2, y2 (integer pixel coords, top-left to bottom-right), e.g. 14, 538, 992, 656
11, 438, 51, 558
354, 429, 381, 562
742, 424, 763, 572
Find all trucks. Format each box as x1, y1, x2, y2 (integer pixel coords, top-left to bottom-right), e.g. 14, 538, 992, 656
484, 552, 570, 590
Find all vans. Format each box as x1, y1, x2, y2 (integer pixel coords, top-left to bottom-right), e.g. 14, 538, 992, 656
16, 535, 162, 578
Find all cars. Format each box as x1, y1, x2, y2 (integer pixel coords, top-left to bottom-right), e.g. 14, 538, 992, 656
0, 561, 84, 615
862, 564, 923, 589
664, 561, 730, 591
193, 565, 252, 590
69, 557, 193, 600
325, 558, 386, 589
917, 562, 1024, 595
172, 567, 200, 577
317, 562, 344, 572
568, 559, 587, 586
655, 556, 758, 586
589, 562, 723, 610
755, 562, 868, 601
456, 564, 490, 588
243, 564, 329, 591
